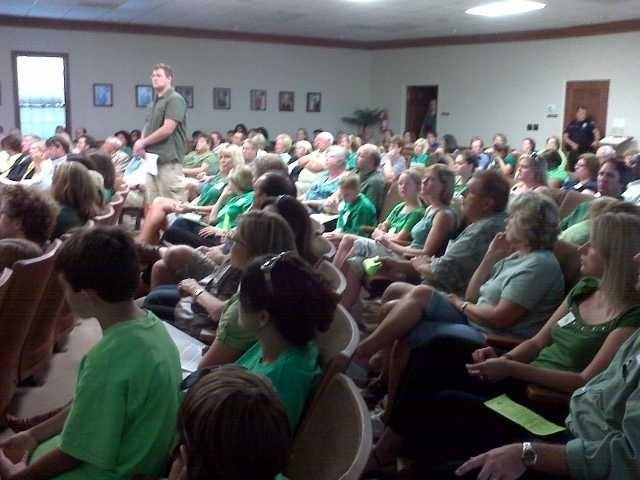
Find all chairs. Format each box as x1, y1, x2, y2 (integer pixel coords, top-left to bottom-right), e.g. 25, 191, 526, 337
285, 303, 361, 444
109, 193, 127, 231
556, 189, 595, 218
0, 268, 15, 438
377, 179, 410, 231
7, 239, 63, 405
275, 372, 373, 480
308, 259, 348, 301
17, 219, 97, 389
437, 237, 582, 350
311, 236, 337, 263
53, 205, 116, 355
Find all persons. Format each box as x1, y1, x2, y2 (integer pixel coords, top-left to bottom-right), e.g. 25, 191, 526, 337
594, 149, 640, 205
140, 194, 310, 333
377, 128, 393, 152
300, 145, 352, 216
486, 143, 516, 177
517, 137, 538, 160
199, 164, 254, 239
454, 252, 640, 480
336, 131, 356, 171
333, 168, 425, 269
0, 228, 184, 480
183, 134, 220, 177
323, 143, 388, 233
295, 128, 311, 142
562, 105, 600, 172
274, 134, 292, 164
362, 169, 510, 411
402, 128, 417, 143
559, 152, 600, 196
132, 62, 189, 214
293, 131, 334, 198
435, 133, 460, 157
483, 133, 508, 159
141, 154, 289, 294
0, 123, 143, 265
149, 170, 299, 292
379, 137, 406, 183
505, 152, 554, 214
365, 199, 640, 480
235, 122, 247, 139
287, 140, 312, 183
595, 145, 616, 165
556, 157, 630, 247
351, 190, 566, 434
137, 144, 244, 245
240, 134, 265, 166
213, 131, 244, 154
191, 130, 201, 151
419, 99, 437, 136
4, 251, 338, 444
541, 148, 569, 188
425, 130, 440, 149
408, 137, 430, 168
469, 136, 491, 172
538, 136, 567, 166
332, 163, 459, 310
451, 149, 480, 201
197, 208, 298, 371
168, 363, 290, 480
323, 171, 378, 250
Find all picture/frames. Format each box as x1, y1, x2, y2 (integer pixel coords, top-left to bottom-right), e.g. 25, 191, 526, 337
212, 86, 233, 110
135, 84, 154, 109
249, 88, 268, 111
277, 91, 294, 112
176, 85, 194, 110
92, 82, 114, 107
305, 91, 321, 112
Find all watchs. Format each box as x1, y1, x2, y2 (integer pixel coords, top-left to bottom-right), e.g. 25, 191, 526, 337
460, 300, 471, 312
520, 441, 538, 469
193, 288, 205, 302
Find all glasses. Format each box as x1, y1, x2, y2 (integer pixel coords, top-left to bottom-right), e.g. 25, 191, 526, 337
260, 251, 286, 296
